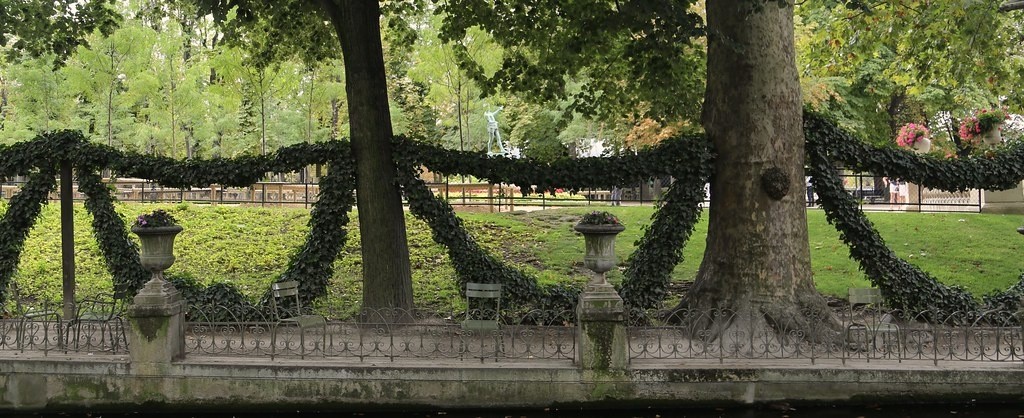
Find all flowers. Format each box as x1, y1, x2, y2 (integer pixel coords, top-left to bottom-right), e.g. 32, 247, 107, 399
895, 122, 928, 148
584, 210, 619, 225
132, 209, 177, 228
957, 105, 1015, 144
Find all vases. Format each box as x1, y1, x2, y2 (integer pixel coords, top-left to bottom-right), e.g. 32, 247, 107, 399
911, 138, 931, 155
980, 123, 1003, 146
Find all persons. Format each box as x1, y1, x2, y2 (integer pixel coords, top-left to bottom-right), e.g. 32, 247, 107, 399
805, 176, 814, 207
894, 184, 900, 203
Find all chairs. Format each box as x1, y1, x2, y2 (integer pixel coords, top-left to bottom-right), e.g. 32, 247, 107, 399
272, 281, 326, 360
460, 282, 505, 363
848, 285, 901, 363
10, 279, 64, 354
65, 284, 129, 354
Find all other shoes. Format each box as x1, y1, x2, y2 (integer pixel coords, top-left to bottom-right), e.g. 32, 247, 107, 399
812, 204, 814, 206
806, 204, 811, 207
